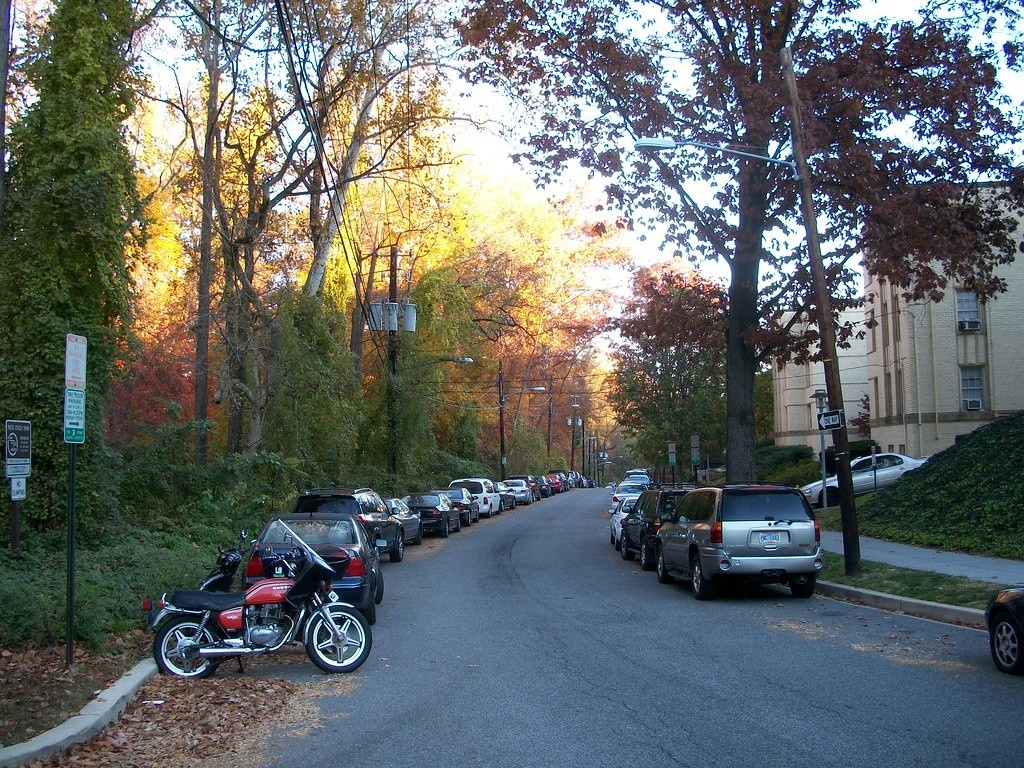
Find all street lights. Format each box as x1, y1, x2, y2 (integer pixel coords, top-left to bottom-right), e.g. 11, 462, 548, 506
632, 46, 864, 578
497, 358, 546, 482
808, 389, 832, 509
385, 355, 474, 500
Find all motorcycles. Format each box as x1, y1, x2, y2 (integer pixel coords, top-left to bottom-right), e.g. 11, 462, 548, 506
198, 526, 351, 647
143, 518, 373, 680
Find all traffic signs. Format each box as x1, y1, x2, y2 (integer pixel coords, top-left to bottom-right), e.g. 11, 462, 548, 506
816, 409, 841, 432
63, 387, 86, 444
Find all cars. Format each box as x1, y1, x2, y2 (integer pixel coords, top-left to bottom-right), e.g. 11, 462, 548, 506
430, 486, 480, 527
401, 491, 463, 539
798, 451, 931, 509
492, 465, 676, 551
242, 512, 385, 628
985, 587, 1024, 679
380, 497, 424, 547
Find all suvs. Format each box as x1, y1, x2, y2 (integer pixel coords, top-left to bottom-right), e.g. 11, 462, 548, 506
289, 487, 406, 563
652, 480, 825, 603
620, 481, 711, 572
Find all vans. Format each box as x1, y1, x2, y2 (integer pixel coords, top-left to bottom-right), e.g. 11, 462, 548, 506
447, 476, 503, 518
